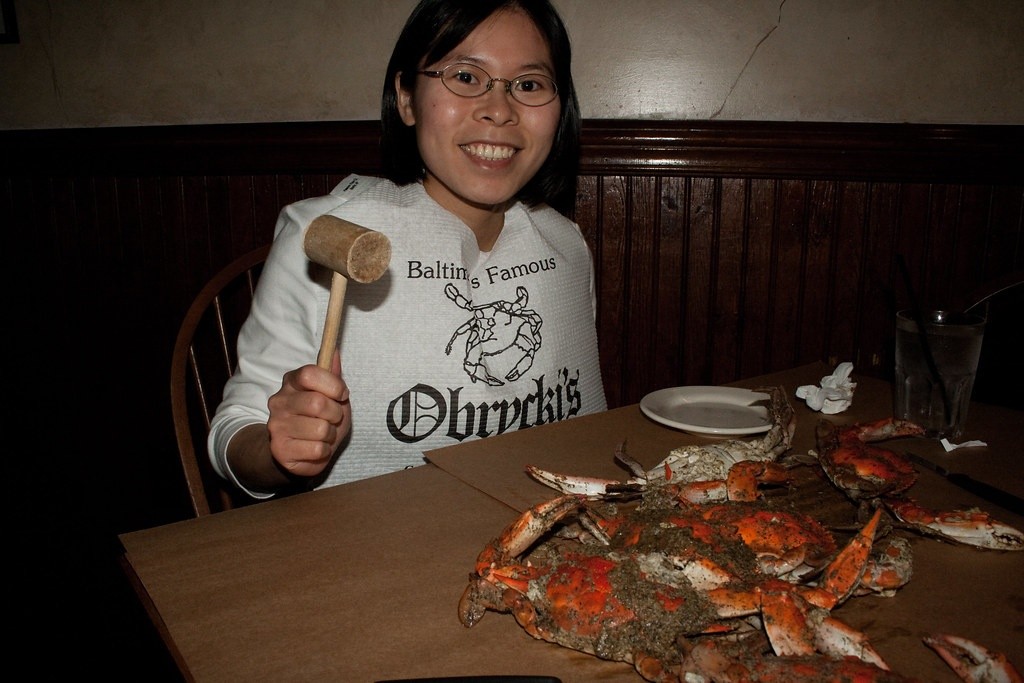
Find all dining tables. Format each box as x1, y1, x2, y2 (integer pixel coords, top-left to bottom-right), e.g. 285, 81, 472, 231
115, 354, 1024, 683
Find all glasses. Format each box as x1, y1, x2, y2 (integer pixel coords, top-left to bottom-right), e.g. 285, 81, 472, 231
418, 63, 563, 107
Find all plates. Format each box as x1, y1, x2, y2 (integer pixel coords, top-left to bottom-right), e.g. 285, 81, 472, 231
640, 386, 795, 439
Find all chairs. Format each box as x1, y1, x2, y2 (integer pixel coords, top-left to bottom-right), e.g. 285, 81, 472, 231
164, 238, 287, 518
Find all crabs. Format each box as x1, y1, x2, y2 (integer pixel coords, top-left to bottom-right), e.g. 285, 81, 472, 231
460, 382, 1024, 683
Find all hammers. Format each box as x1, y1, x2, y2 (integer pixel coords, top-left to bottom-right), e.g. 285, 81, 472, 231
304, 214, 392, 368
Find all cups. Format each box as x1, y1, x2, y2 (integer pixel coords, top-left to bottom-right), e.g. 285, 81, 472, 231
893, 308, 987, 442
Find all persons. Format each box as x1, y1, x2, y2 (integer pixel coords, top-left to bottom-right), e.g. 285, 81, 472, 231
206, 0, 609, 501
